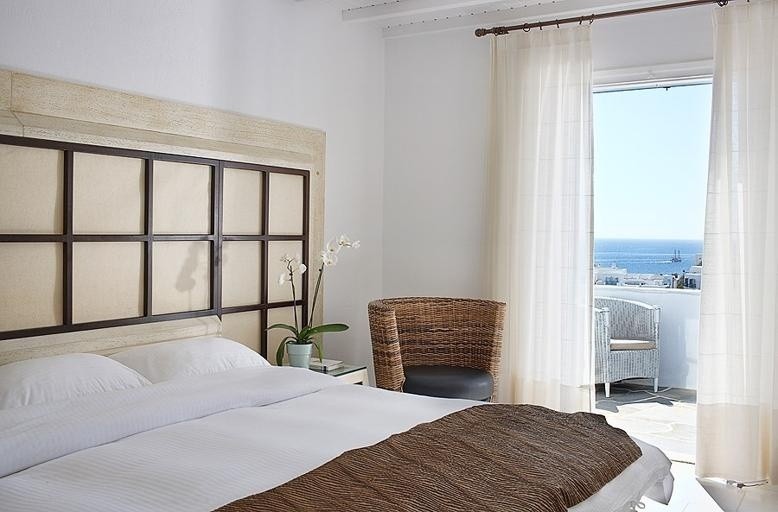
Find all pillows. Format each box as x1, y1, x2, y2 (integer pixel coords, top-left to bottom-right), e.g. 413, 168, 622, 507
0, 333, 272, 414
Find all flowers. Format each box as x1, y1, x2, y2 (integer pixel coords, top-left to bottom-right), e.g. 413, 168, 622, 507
275, 233, 360, 371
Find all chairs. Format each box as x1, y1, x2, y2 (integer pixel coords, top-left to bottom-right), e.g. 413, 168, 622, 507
592, 296, 660, 397
369, 295, 507, 407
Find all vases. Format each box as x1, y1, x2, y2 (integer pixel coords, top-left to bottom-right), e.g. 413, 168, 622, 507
286, 341, 314, 371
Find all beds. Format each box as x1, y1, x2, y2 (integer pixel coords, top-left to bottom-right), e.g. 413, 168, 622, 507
0, 314, 672, 510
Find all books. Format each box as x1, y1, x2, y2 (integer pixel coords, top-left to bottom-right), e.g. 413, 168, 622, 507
309, 357, 345, 371
